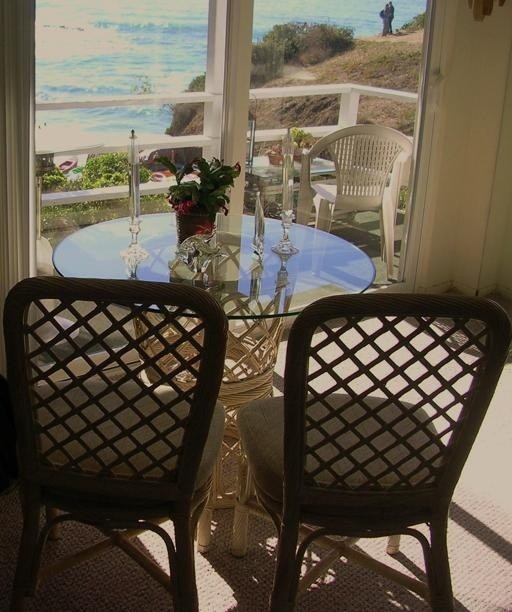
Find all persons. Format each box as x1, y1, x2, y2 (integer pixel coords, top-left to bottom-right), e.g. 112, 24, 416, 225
383, 4, 389, 35
388, 2, 394, 33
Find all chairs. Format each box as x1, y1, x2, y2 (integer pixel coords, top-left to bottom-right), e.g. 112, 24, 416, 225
306, 121, 415, 278
5, 278, 228, 611
235, 292, 511, 612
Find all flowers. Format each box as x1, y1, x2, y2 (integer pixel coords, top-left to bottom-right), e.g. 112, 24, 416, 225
264, 127, 316, 156
149, 153, 241, 215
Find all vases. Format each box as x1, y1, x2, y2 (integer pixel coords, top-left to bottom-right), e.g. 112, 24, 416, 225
268, 151, 303, 164
174, 207, 218, 243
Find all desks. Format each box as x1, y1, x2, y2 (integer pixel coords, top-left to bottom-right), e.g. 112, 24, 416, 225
241, 153, 336, 218
51, 211, 376, 509
